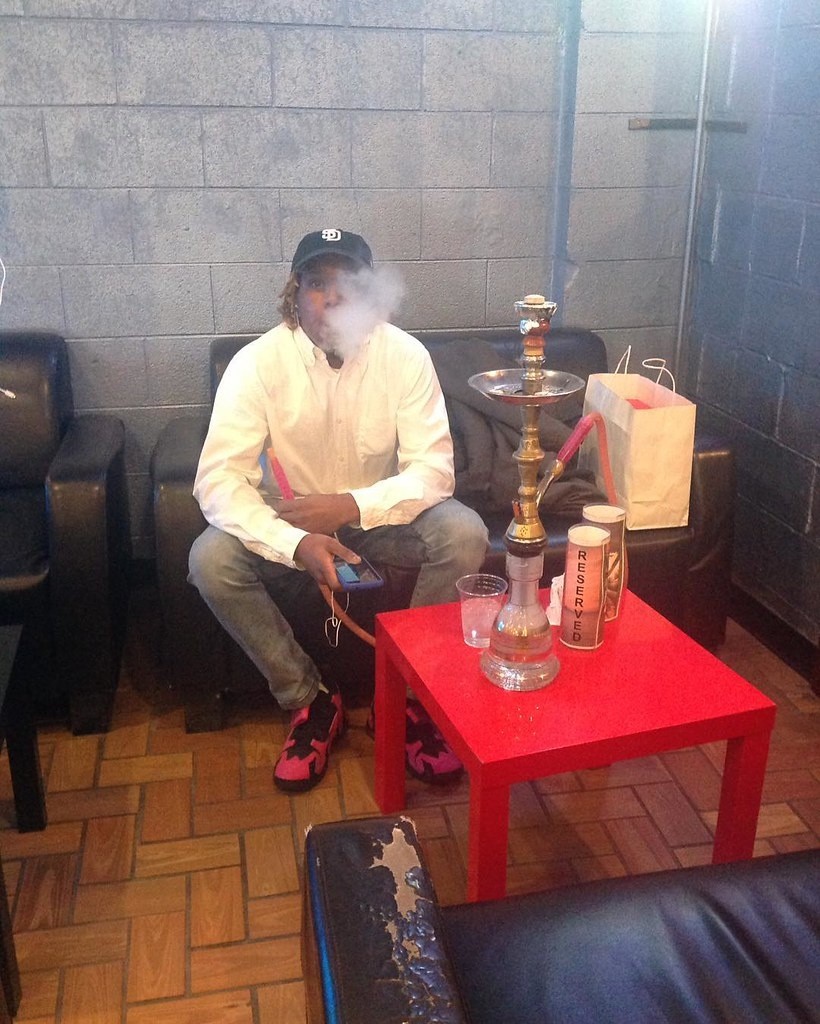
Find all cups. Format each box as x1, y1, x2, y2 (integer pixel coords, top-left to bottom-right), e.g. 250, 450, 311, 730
456, 574, 508, 649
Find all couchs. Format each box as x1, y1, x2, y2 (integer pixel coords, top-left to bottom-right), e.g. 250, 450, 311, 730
0, 329, 129, 735
296, 813, 820, 1024
150, 325, 735, 734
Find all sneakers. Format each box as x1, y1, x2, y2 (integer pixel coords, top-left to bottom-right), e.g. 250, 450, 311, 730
273, 679, 348, 792
366, 695, 464, 783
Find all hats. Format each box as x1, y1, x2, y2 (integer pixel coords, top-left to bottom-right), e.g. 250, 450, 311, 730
290, 230, 378, 278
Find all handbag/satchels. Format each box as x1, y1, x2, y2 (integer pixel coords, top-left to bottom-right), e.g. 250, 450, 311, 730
577, 347, 698, 532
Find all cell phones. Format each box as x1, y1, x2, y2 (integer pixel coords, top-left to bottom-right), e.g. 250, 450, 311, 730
333, 555, 385, 592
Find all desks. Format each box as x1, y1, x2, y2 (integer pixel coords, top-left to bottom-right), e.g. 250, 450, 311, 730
372, 581, 779, 902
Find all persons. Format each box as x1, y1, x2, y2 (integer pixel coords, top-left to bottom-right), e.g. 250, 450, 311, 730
187, 230, 490, 789
